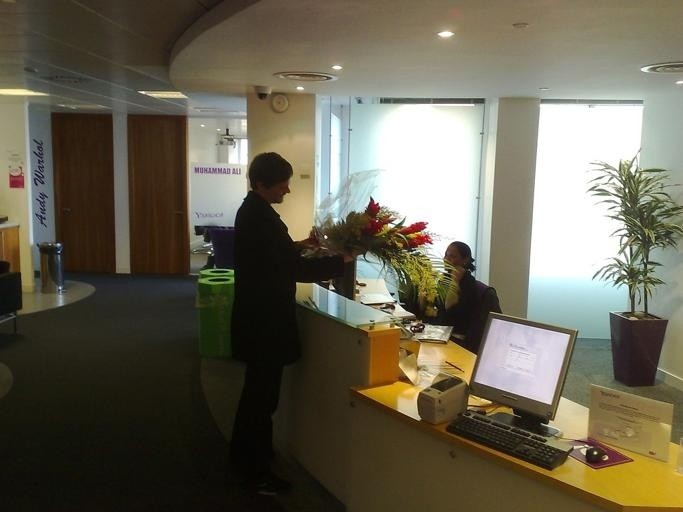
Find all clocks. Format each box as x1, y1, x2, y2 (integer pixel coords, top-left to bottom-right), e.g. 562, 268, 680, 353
271, 95, 289, 113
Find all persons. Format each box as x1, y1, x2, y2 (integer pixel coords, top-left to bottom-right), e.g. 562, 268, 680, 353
227, 151, 357, 496
430, 241, 503, 353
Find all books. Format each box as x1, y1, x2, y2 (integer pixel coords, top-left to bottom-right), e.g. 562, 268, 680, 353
415, 324, 454, 343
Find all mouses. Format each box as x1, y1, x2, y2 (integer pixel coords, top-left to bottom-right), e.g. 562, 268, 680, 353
585, 447, 606, 463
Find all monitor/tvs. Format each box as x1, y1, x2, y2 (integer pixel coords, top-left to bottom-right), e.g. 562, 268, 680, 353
468, 311, 580, 439
330, 258, 357, 301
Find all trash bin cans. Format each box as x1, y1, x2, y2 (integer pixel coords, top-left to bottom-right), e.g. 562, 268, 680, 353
36, 242, 69, 294
197, 268, 235, 359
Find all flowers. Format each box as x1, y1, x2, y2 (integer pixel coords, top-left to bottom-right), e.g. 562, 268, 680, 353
300, 194, 461, 319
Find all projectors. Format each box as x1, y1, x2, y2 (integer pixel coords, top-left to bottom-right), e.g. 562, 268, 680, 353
218, 135, 236, 146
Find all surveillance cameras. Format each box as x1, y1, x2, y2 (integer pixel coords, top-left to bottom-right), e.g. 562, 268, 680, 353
254, 86, 272, 102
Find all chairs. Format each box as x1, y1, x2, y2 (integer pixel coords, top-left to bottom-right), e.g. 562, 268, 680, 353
0, 260, 24, 337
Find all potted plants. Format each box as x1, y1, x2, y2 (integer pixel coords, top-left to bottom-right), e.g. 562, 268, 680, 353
583, 146, 683, 388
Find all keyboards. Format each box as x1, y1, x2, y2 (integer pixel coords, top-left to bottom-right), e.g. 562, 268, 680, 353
446, 409, 575, 471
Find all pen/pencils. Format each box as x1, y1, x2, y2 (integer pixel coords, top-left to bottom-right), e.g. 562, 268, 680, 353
445, 361, 465, 372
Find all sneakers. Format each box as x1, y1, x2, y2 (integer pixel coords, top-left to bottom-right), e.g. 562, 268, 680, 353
228, 440, 294, 497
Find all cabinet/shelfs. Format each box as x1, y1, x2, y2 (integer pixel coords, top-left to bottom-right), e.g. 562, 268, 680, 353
0, 222, 22, 273
